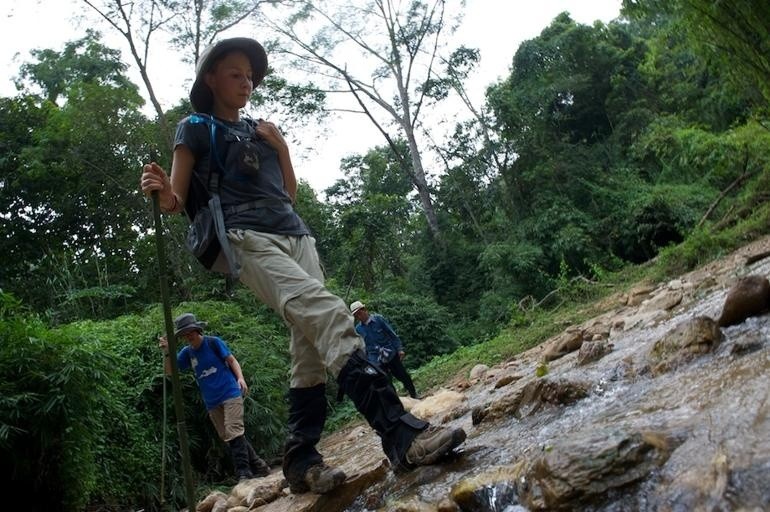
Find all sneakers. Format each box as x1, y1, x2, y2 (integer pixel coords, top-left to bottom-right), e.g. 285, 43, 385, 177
289, 461, 346, 494
388, 414, 466, 475
238, 467, 271, 483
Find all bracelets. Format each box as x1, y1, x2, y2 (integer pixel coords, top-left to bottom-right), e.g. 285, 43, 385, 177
163, 352, 169, 357
166, 193, 177, 212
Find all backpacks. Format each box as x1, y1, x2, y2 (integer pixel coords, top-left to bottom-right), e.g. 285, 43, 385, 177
187, 112, 259, 275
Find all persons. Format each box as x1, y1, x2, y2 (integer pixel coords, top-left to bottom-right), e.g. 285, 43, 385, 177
350, 301, 425, 400
140, 36, 467, 496
158, 313, 270, 484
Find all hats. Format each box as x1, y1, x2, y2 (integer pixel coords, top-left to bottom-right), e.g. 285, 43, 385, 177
173, 313, 207, 337
190, 38, 267, 113
350, 301, 365, 316
